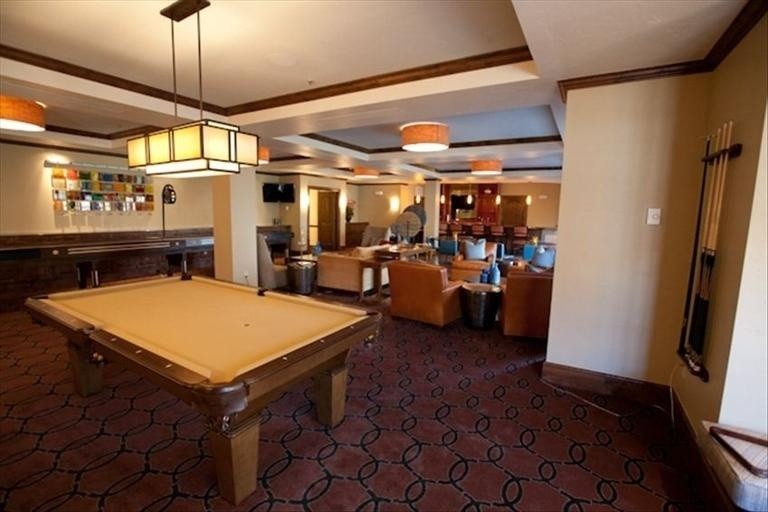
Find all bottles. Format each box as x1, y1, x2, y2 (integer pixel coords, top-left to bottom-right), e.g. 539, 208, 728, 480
480, 262, 501, 284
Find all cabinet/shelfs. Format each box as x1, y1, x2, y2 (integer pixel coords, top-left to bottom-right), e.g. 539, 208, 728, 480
44, 160, 156, 217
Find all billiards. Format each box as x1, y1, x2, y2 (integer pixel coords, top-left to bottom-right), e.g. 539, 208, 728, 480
366, 333, 374, 341
92, 351, 104, 361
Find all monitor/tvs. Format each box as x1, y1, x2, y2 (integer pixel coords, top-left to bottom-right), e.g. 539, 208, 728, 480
263, 183, 296, 202
451, 195, 475, 210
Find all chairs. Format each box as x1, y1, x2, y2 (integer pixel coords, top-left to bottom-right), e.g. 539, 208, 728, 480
439, 221, 528, 254
451, 241, 497, 284
257, 233, 288, 289
439, 239, 459, 264
387, 260, 463, 327
501, 268, 553, 339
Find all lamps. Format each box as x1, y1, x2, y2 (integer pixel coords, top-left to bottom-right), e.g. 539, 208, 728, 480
470, 160, 502, 175
257, 146, 270, 165
415, 182, 531, 205
354, 168, 378, 179
399, 121, 450, 152
161, 183, 176, 236
126, 0, 258, 179
0, 94, 46, 132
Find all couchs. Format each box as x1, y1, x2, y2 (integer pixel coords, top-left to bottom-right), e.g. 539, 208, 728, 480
316, 251, 394, 299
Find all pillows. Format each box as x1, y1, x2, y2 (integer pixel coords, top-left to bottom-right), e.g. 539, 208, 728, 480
464, 238, 486, 260
531, 245, 555, 268
352, 243, 391, 259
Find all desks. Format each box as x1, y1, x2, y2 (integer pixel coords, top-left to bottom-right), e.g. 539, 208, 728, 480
68, 230, 295, 290
288, 261, 316, 295
461, 284, 502, 330
375, 247, 436, 292
25, 272, 384, 506
358, 257, 394, 304
290, 254, 318, 266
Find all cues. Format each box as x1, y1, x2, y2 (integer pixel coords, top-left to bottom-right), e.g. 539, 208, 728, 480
678, 120, 734, 372
